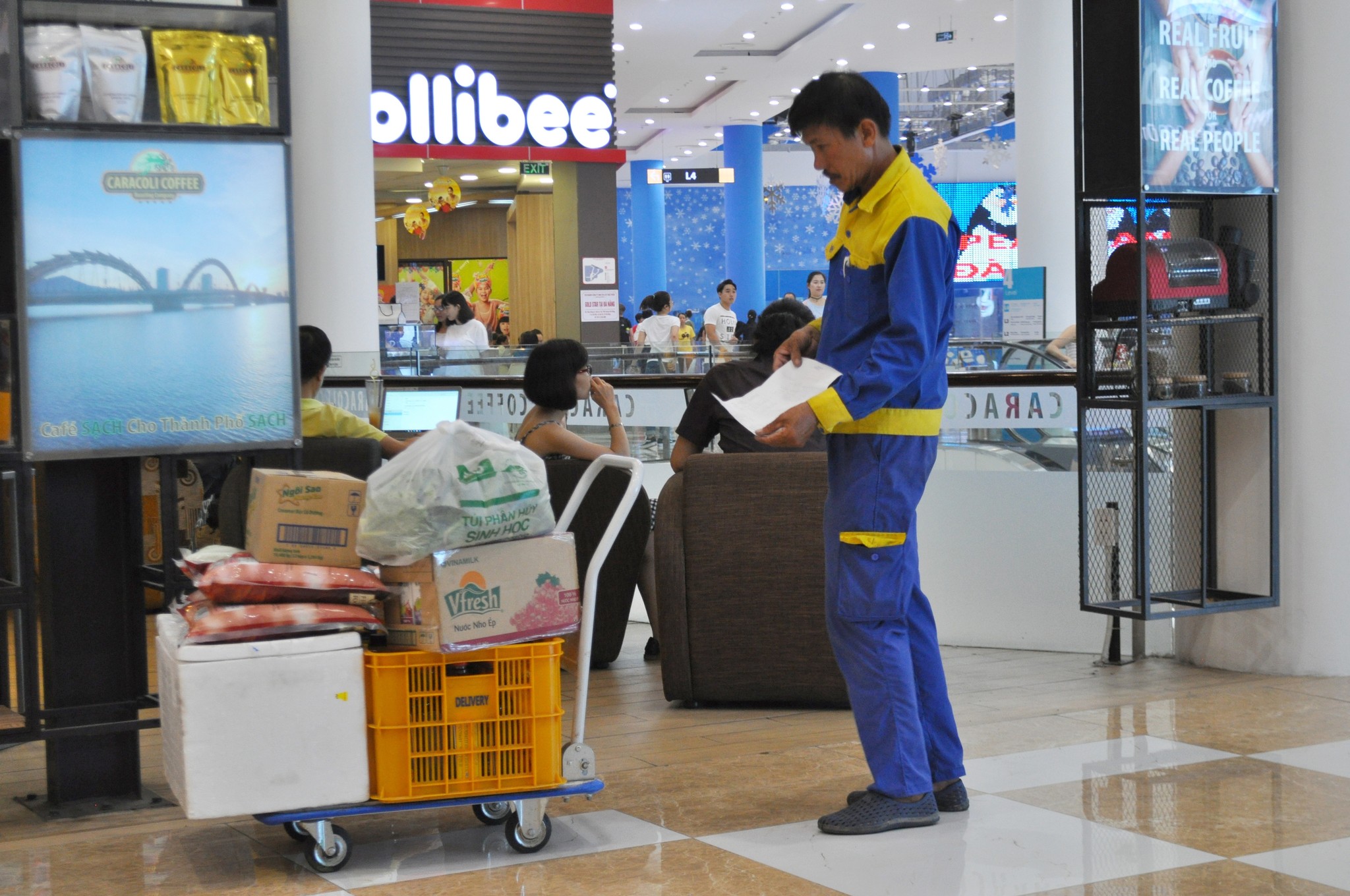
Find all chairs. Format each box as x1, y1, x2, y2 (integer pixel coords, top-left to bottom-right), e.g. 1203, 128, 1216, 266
212, 429, 389, 553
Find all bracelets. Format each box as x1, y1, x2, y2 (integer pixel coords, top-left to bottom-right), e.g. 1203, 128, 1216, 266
631, 365, 637, 369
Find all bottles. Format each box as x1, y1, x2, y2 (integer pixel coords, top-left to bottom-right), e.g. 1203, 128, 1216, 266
1223, 373, 1254, 394
1176, 373, 1212, 396
1129, 332, 1175, 400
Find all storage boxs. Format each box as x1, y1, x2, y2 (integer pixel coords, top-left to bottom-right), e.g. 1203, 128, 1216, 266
242, 461, 371, 573
379, 528, 589, 656
361, 639, 570, 798
148, 607, 373, 823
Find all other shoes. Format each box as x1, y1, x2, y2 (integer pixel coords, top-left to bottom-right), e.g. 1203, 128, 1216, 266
818, 793, 939, 834
643, 637, 660, 663
846, 780, 969, 812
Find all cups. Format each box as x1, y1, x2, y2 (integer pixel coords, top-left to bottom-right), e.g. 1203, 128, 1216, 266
365, 379, 385, 430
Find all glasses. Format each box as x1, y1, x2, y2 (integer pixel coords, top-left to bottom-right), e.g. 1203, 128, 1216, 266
576, 364, 592, 376
668, 301, 674, 306
433, 307, 445, 312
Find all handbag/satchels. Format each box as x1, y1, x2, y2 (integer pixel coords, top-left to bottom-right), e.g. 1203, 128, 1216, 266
378, 303, 406, 325
354, 421, 556, 565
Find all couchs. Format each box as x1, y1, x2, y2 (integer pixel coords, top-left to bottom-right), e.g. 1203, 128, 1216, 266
536, 455, 653, 670
639, 442, 850, 717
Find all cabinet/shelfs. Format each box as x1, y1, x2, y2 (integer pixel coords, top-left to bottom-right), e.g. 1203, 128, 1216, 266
1061, 191, 1288, 622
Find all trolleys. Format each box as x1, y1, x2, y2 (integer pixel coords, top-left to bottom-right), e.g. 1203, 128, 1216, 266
250, 454, 643, 873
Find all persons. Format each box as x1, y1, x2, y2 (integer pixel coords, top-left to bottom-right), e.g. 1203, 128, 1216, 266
430, 294, 448, 376
437, 291, 498, 376
299, 325, 432, 460
631, 291, 681, 374
419, 279, 438, 324
801, 271, 827, 320
754, 69, 970, 835
784, 292, 796, 301
489, 316, 544, 376
452, 262, 511, 344
1045, 324, 1108, 368
514, 338, 663, 662
703, 279, 739, 374
612, 303, 758, 374
1148, 0, 1274, 187
